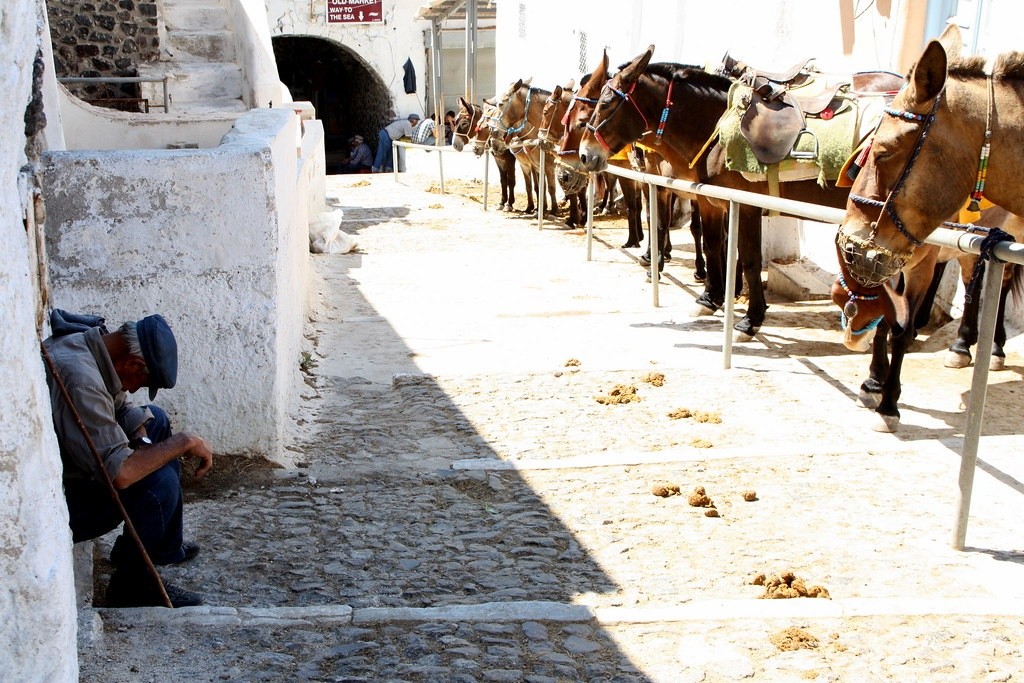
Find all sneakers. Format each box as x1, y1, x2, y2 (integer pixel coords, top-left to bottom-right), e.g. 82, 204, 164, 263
110, 535, 200, 569
105, 570, 205, 609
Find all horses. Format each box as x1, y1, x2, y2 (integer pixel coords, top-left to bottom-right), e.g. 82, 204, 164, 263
451, 44, 906, 342
832, 23, 1024, 433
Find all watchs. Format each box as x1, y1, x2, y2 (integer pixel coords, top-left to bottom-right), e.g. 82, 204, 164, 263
130, 435, 154, 451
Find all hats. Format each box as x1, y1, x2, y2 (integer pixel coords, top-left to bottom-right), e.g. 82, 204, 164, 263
136, 314, 178, 401
355, 135, 363, 144
408, 114, 420, 120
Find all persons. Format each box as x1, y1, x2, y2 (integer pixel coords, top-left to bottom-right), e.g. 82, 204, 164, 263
444, 111, 457, 145
370, 113, 422, 173
411, 112, 441, 146
342, 135, 374, 173
40, 308, 215, 609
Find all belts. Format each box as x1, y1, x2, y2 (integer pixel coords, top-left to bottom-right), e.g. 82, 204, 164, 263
383, 128, 389, 135
